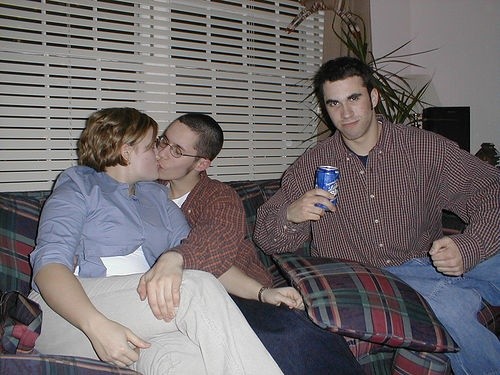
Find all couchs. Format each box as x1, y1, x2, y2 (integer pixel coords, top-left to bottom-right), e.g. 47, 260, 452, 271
0, 177, 500, 375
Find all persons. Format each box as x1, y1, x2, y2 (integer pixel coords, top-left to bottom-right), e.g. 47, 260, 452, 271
136, 113, 367, 375
27, 105, 306, 375
253, 56, 500, 375
258, 286, 269, 303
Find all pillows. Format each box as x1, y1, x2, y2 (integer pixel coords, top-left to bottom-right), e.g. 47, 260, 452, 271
273, 249, 461, 354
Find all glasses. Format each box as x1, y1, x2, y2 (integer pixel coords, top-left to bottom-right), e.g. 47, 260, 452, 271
157, 135, 207, 160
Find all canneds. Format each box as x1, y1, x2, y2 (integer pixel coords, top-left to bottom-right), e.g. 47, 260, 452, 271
314, 166, 339, 211
